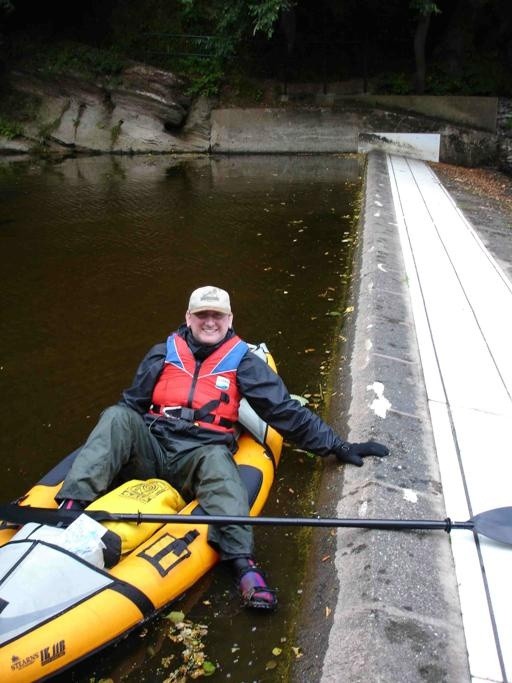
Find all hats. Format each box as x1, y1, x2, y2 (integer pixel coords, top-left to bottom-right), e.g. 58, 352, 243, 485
189, 285, 231, 315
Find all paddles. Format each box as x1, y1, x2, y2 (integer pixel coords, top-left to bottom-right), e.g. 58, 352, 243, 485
0, 503, 512, 545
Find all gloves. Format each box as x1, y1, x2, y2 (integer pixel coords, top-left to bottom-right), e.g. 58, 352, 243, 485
334, 441, 390, 467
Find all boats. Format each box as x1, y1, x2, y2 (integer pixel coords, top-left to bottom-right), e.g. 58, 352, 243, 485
1, 344, 285, 682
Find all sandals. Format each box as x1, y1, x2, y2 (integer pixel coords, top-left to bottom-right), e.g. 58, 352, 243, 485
232, 564, 281, 611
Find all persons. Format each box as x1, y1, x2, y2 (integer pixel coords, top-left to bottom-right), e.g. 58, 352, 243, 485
54, 284, 389, 610
